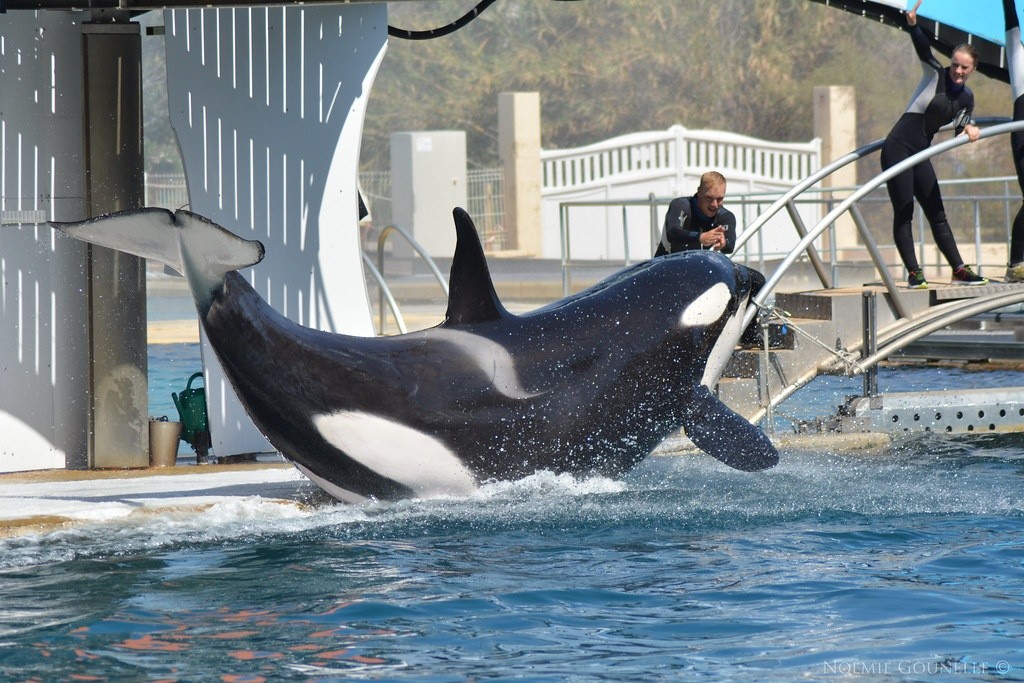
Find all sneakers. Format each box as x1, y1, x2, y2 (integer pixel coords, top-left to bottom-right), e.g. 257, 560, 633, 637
1004, 262, 1024, 281
951, 265, 988, 285
908, 269, 926, 289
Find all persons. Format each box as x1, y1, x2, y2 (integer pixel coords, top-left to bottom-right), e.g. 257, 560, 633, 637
880, 1, 990, 290
654, 172, 782, 349
1003, 0, 1024, 284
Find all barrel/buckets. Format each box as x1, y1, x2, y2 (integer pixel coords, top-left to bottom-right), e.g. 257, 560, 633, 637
148, 421, 182, 467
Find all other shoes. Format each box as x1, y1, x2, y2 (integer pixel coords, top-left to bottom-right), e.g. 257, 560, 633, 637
743, 323, 781, 349
